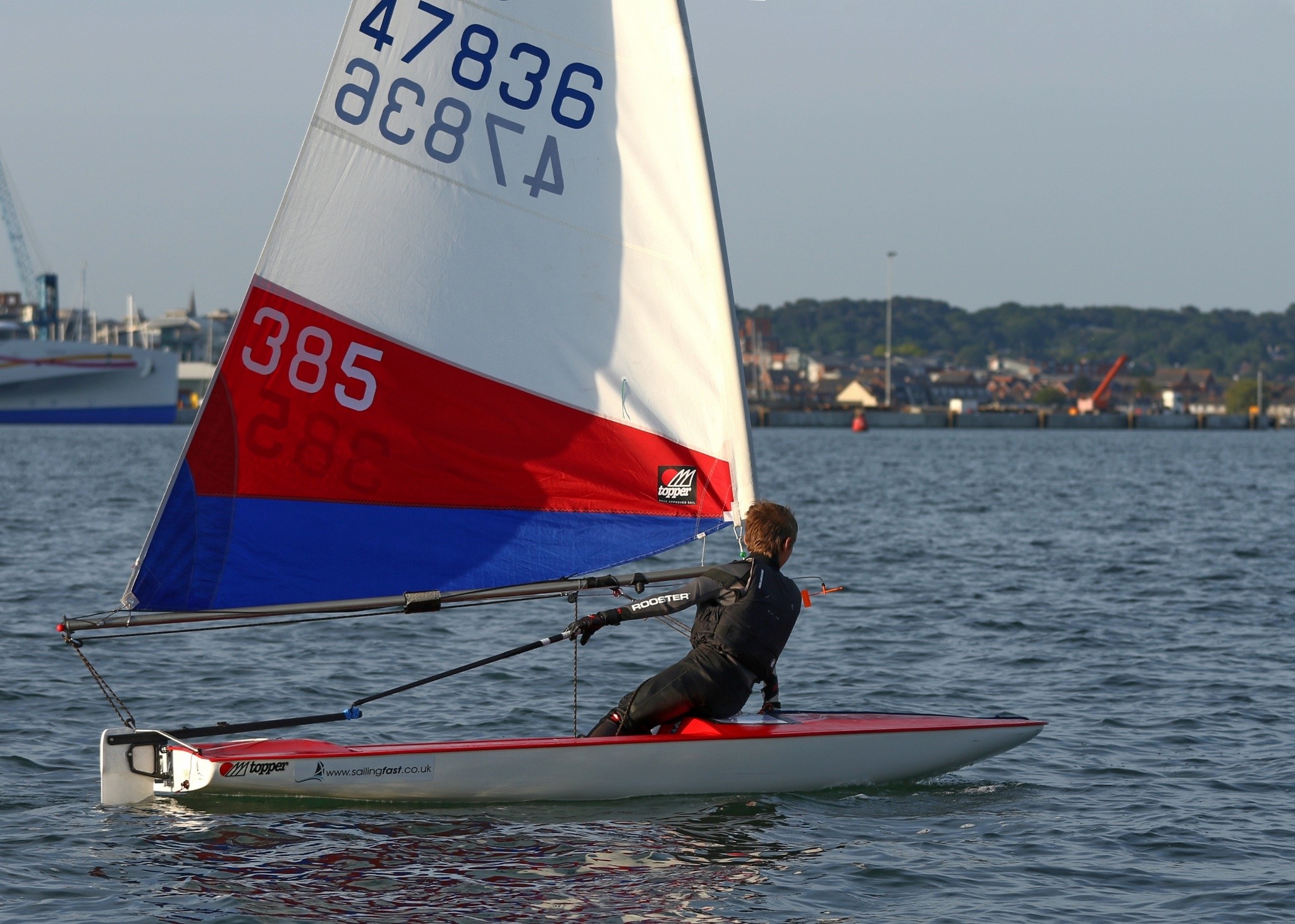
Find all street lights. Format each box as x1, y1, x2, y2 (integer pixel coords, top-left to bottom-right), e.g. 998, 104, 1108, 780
887, 250, 902, 400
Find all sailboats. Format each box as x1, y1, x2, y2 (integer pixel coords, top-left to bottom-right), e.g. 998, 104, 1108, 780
46, 0, 1053, 817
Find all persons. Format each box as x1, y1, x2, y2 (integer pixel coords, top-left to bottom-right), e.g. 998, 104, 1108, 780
565, 498, 803, 739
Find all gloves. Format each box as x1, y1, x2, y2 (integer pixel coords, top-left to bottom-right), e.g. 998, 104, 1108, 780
563, 614, 604, 646
